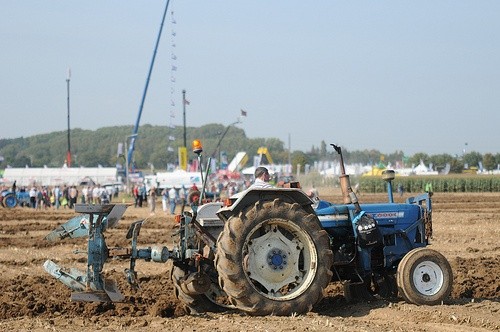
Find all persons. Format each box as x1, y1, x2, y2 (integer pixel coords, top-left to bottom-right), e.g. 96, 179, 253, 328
250, 167, 314, 198
12, 178, 254, 216
311, 181, 433, 199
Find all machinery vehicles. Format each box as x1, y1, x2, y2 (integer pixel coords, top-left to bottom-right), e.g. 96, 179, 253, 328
0, 181, 30, 208
42, 143, 454, 318
